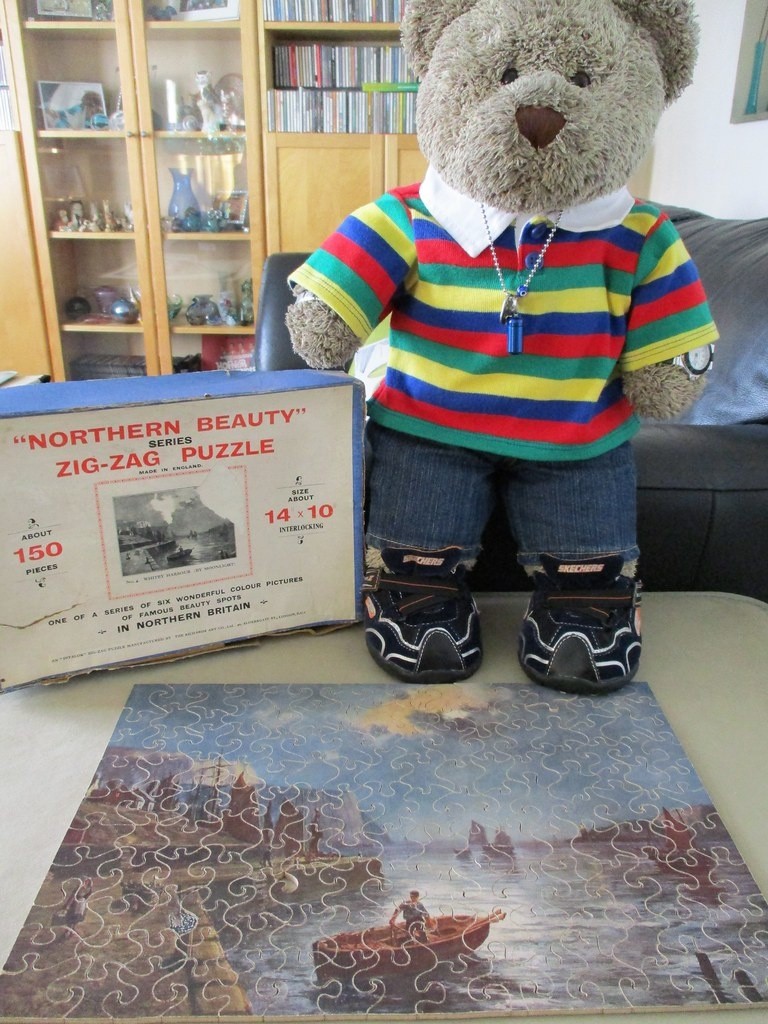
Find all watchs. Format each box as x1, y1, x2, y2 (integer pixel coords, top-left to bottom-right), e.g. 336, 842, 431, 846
658, 344, 715, 381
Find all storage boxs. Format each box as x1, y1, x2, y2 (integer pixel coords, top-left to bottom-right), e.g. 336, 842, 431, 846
0, 370, 367, 690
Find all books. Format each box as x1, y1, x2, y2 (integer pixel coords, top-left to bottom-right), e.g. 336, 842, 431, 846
264, 0, 409, 23
267, 41, 422, 134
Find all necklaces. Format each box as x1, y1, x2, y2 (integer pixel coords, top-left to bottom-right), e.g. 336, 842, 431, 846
480, 202, 564, 356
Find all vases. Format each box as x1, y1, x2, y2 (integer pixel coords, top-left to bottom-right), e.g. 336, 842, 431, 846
168, 167, 200, 230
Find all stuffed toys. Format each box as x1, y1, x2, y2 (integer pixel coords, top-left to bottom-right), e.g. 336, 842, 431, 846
284, 0, 720, 694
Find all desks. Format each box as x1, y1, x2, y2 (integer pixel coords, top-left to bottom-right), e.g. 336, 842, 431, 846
0, 591, 768, 1024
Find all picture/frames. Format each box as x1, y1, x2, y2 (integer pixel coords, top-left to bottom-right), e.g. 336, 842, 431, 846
37, 81, 108, 130
37, 0, 94, 18
209, 190, 249, 225
169, 0, 239, 23
45, 164, 86, 198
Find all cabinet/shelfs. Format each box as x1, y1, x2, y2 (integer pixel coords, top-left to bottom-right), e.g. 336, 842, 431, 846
257, 1, 430, 252
6, 0, 264, 386
0, 0, 53, 380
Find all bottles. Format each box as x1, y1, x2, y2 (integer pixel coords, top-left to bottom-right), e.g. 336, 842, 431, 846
94, 285, 116, 315
219, 291, 236, 321
186, 294, 218, 326
160, 166, 230, 233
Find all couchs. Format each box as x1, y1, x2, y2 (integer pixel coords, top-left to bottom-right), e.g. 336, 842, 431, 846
255, 197, 768, 601
